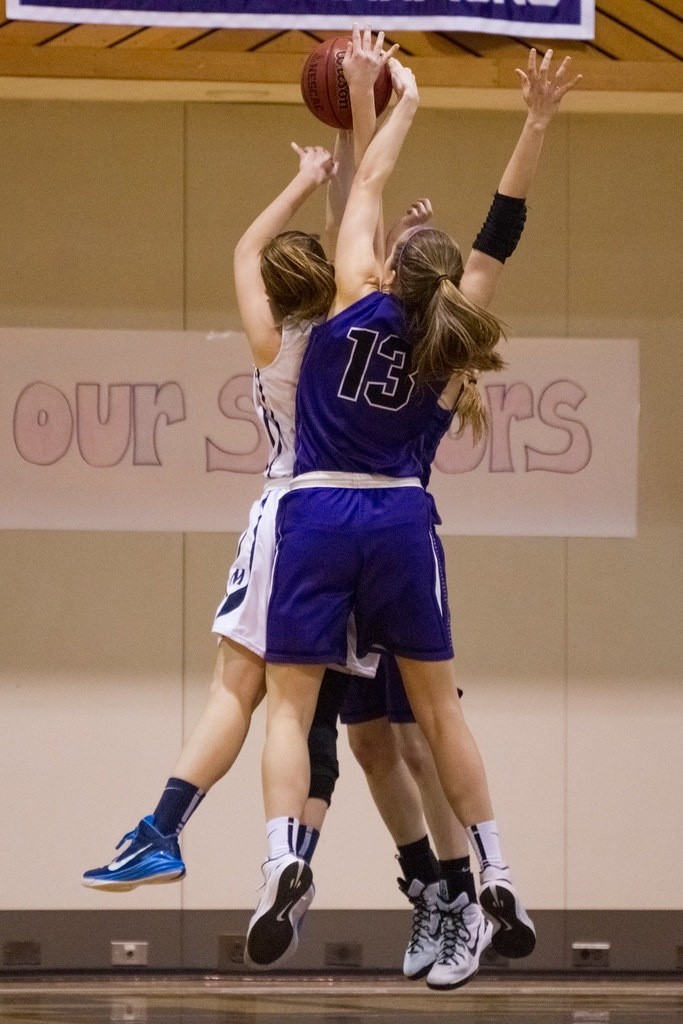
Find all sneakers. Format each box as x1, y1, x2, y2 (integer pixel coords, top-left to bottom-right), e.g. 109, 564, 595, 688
397, 878, 443, 979
478, 865, 536, 959
425, 891, 494, 990
82, 815, 186, 893
244, 884, 316, 971
247, 853, 313, 964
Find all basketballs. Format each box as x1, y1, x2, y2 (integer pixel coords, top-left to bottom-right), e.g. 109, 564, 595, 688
300, 36, 393, 130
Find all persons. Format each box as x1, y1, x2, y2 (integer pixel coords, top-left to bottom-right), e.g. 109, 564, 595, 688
78, 22, 399, 927
240, 48, 584, 969
297, 129, 494, 990
